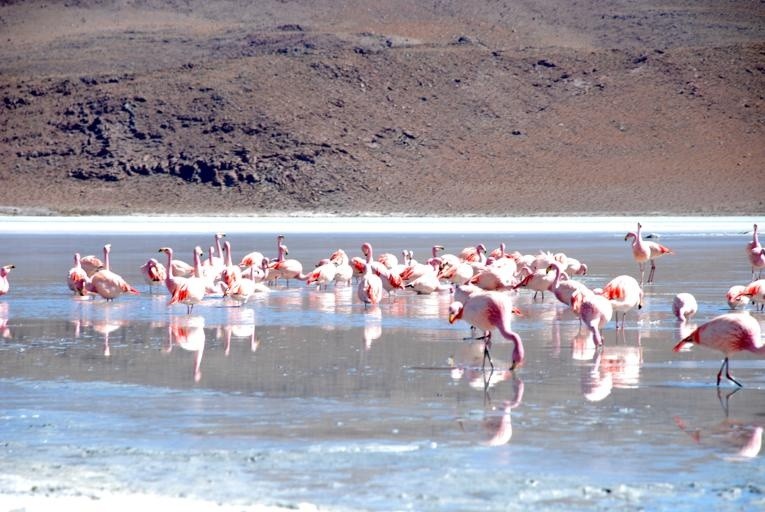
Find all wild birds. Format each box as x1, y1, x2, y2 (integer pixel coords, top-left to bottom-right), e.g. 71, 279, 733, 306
746, 223, 765, 277
673, 388, 765, 464
90, 307, 124, 357
0, 301, 14, 339
225, 306, 261, 359
455, 369, 525, 447
671, 293, 699, 323
700, 312, 764, 386
0, 265, 17, 299
358, 317, 382, 350
163, 310, 208, 384
726, 279, 765, 311
62, 223, 669, 371
577, 329, 643, 403
675, 322, 699, 355
